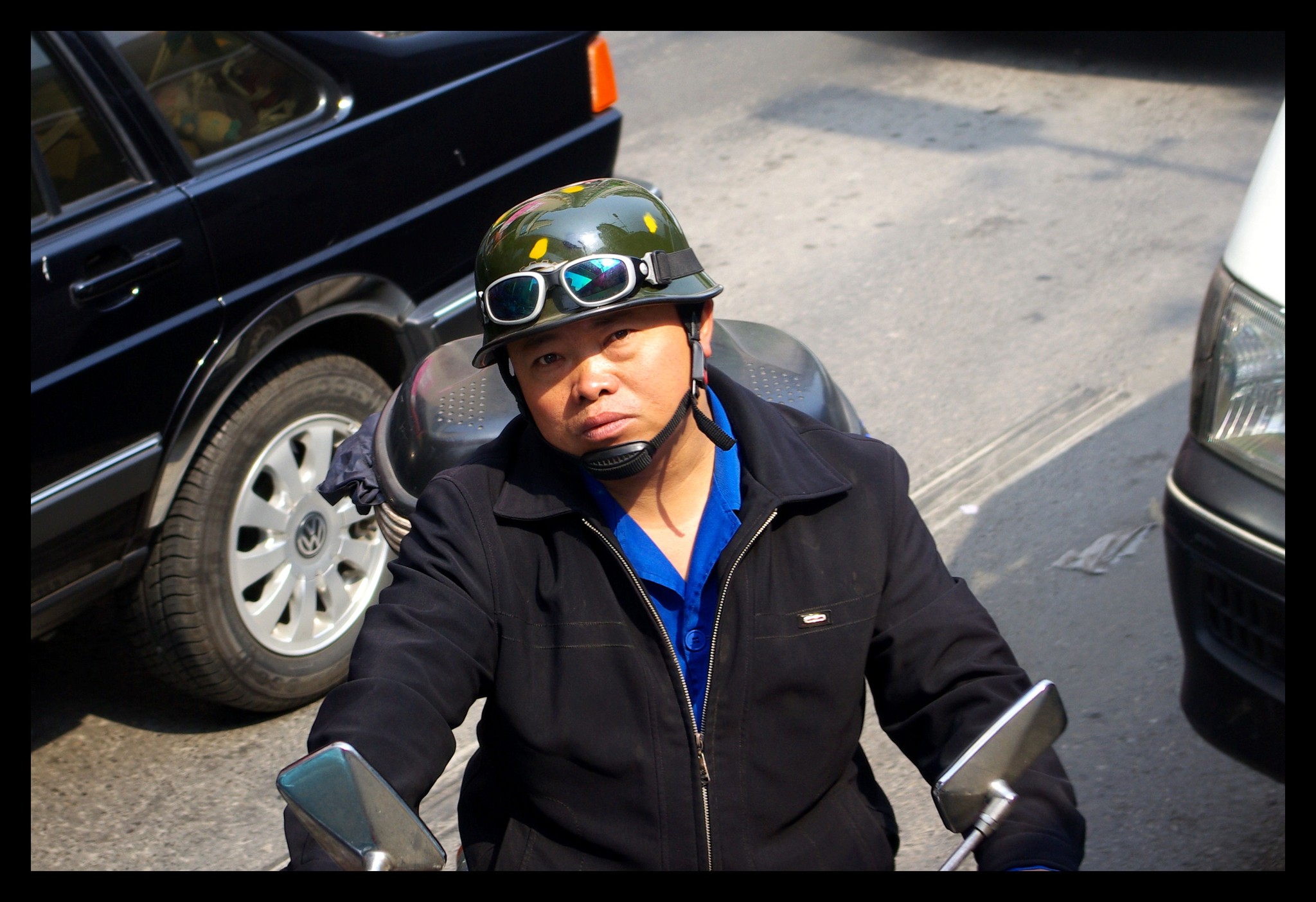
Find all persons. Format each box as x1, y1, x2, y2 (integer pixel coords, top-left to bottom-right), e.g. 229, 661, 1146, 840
306, 178, 1087, 874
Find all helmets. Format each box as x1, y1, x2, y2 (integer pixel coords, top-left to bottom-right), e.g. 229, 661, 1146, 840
470, 177, 722, 370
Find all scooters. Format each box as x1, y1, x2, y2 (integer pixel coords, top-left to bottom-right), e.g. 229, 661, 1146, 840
272, 316, 1070, 874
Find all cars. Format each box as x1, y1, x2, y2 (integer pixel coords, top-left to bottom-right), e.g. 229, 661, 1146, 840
1161, 95, 1286, 722
30, 24, 625, 727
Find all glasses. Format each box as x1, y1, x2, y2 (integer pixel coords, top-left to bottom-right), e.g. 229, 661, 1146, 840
478, 252, 649, 327
